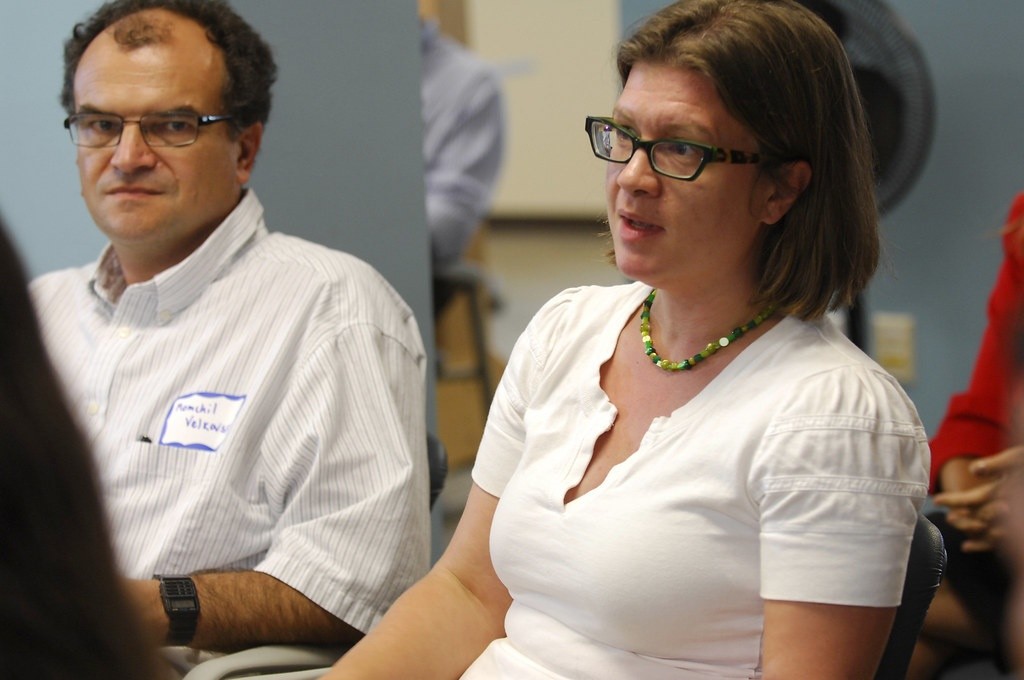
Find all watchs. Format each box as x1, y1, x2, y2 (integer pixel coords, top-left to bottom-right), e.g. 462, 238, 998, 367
153, 574, 200, 644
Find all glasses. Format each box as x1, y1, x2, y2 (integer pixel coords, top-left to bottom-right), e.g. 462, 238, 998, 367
584, 114, 775, 182
63, 112, 235, 148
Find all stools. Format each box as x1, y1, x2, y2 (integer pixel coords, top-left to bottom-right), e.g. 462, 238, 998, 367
430, 265, 493, 415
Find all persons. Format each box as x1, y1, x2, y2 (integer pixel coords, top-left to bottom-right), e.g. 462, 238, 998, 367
420, 15, 507, 312
0, 216, 164, 680
315, 1, 929, 680
910, 192, 1024, 680
28, 0, 432, 654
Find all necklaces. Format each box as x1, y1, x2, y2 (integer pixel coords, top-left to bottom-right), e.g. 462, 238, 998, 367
641, 289, 774, 370
